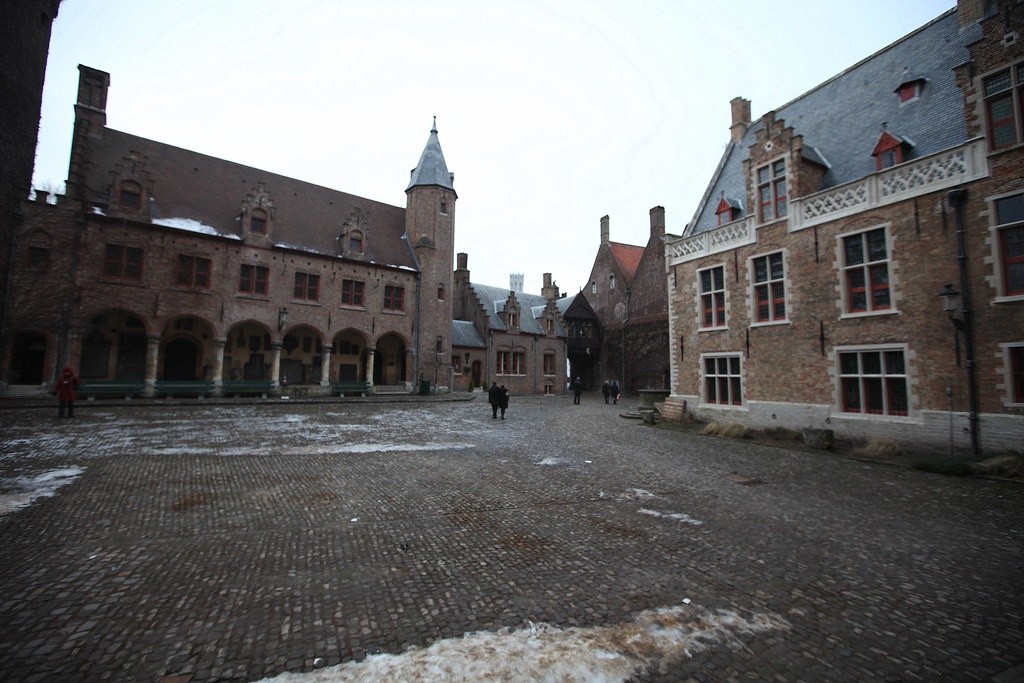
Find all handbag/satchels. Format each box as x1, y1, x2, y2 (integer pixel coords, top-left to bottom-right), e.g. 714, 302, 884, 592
617, 394, 620, 400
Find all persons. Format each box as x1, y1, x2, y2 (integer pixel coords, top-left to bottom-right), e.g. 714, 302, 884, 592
574, 377, 582, 404
489, 382, 509, 419
53, 368, 80, 417
602, 381, 619, 404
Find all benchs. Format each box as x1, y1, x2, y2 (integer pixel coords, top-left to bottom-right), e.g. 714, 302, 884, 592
223, 380, 276, 399
155, 380, 215, 400
75, 378, 145, 400
332, 381, 372, 398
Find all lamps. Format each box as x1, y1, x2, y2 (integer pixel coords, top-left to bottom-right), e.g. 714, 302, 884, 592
278, 306, 288, 330
938, 283, 964, 329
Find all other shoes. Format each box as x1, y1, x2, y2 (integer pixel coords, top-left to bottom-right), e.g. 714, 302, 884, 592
502, 417, 504, 419
493, 417, 497, 419
